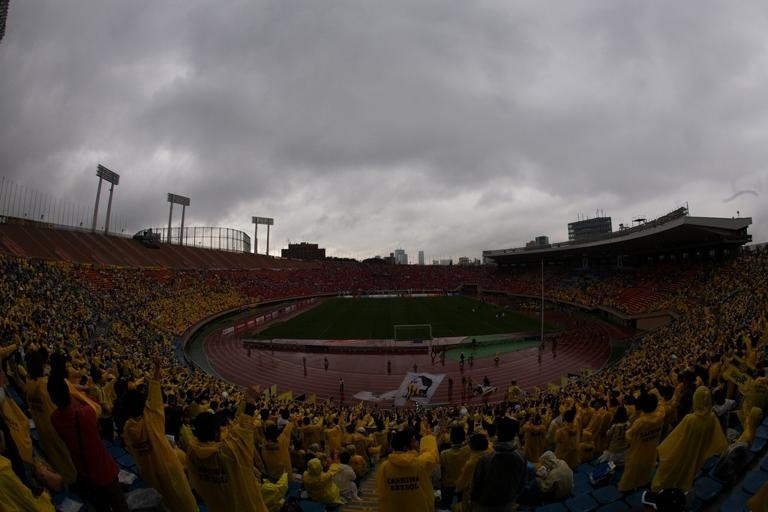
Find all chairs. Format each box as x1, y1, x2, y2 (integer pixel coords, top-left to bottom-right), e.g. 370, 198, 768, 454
52, 491, 89, 512
526, 412, 768, 511
100, 439, 144, 492
262, 473, 326, 512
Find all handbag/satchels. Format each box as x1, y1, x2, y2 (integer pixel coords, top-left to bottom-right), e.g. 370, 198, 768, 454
715, 441, 748, 483
587, 462, 616, 489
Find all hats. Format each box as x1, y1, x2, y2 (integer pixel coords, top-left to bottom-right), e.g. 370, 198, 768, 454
641, 488, 686, 512
190, 412, 225, 432
